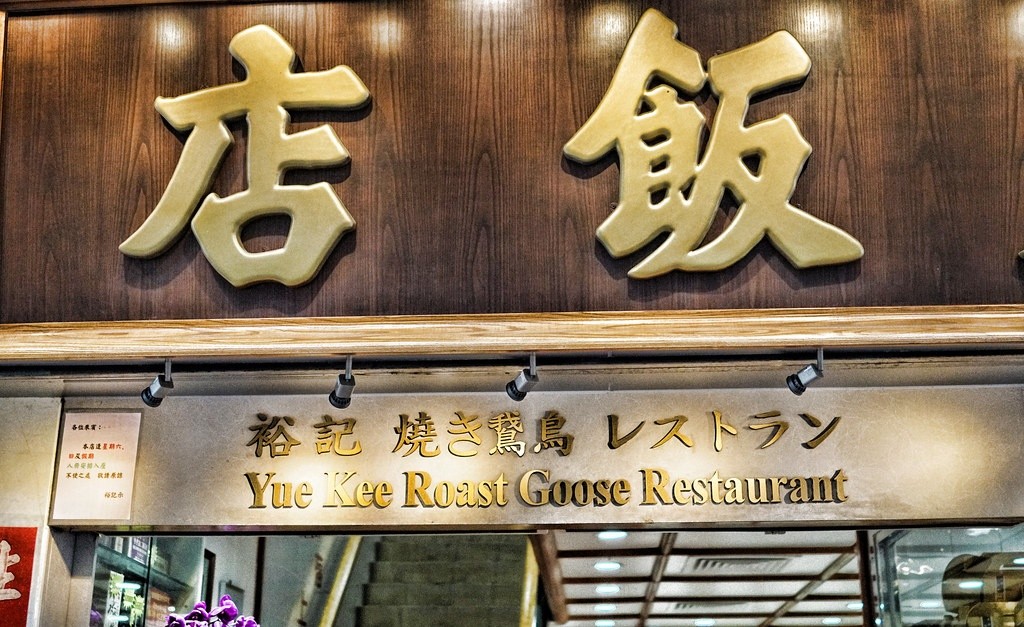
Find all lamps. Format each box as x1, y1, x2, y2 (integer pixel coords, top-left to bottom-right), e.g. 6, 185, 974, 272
506, 351, 539, 401
786, 345, 824, 396
140, 358, 174, 408
328, 353, 356, 409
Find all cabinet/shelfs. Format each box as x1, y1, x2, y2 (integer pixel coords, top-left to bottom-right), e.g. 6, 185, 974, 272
66, 529, 205, 627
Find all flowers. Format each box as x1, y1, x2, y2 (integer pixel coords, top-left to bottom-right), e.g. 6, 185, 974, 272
165, 595, 259, 627
